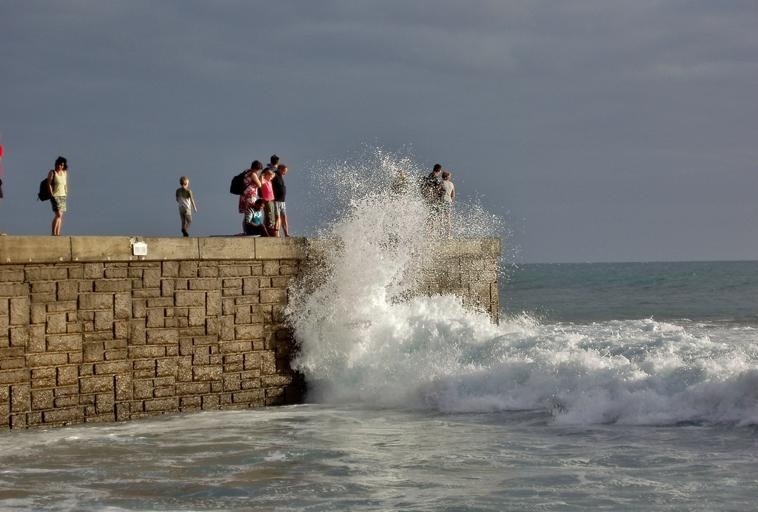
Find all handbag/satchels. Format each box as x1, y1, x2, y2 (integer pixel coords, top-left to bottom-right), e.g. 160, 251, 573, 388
38, 169, 55, 200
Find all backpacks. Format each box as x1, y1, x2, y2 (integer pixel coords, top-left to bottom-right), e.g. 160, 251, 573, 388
230, 170, 256, 194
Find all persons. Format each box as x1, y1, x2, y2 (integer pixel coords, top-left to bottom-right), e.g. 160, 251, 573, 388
239, 159, 262, 214
274, 164, 292, 237
425, 164, 442, 205
176, 176, 197, 236
436, 170, 456, 239
264, 155, 280, 172
242, 199, 270, 237
393, 170, 409, 195
47, 155, 68, 237
260, 168, 276, 228
0, 144, 4, 200
